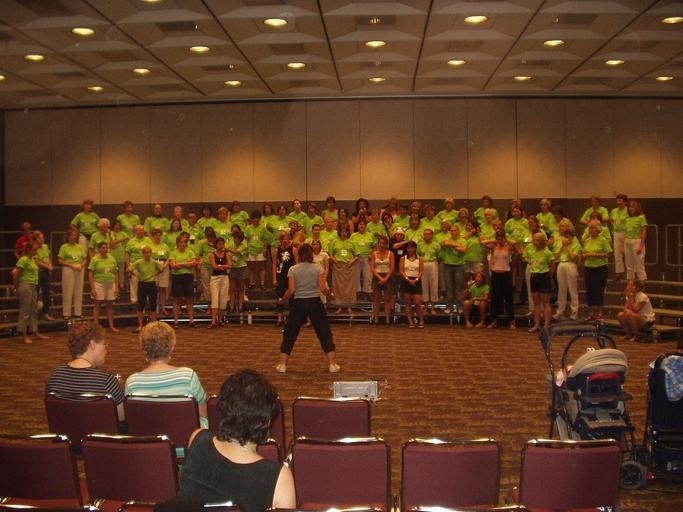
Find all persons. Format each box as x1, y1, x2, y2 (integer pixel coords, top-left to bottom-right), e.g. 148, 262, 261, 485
57, 194, 655, 344
179, 367, 297, 512
11, 222, 53, 343
125, 322, 210, 431
276, 244, 340, 373
44, 318, 126, 435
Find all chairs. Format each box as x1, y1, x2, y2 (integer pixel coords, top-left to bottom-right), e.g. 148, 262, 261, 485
124, 393, 201, 463
207, 395, 287, 460
511, 437, 624, 512
45, 391, 119, 442
401, 438, 501, 512
0, 432, 89, 510
81, 433, 179, 512
291, 442, 390, 511
183, 439, 280, 464
292, 396, 372, 440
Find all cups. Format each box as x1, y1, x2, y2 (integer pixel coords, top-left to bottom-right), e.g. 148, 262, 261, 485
677, 316, 683, 328
452, 305, 457, 312
255, 307, 259, 312
659, 272, 666, 281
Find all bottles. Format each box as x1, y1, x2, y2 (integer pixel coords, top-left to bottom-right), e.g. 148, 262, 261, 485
652, 325, 658, 343
247, 310, 252, 325
239, 311, 243, 324
349, 315, 353, 328
466, 280, 476, 288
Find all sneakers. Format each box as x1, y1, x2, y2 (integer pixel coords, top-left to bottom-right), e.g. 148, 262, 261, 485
525, 312, 578, 333
431, 307, 464, 315
329, 364, 340, 372
466, 320, 521, 330
276, 364, 286, 373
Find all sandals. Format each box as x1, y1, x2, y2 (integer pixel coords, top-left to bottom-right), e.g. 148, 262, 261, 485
174, 320, 228, 329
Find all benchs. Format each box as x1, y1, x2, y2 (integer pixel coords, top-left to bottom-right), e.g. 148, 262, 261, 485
1, 265, 683, 342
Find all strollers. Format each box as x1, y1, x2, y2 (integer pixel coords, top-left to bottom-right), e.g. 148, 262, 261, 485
637, 342, 682, 477
526, 312, 652, 493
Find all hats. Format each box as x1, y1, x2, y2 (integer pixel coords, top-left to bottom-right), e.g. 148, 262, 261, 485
393, 227, 405, 238
